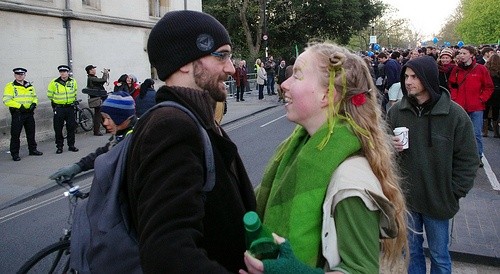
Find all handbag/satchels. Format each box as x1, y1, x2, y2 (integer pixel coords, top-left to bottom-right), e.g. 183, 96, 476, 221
88, 97, 102, 108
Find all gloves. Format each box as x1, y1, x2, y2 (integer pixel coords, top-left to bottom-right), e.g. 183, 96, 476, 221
48, 164, 81, 181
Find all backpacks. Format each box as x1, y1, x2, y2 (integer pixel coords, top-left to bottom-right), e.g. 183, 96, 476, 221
70, 100, 215, 274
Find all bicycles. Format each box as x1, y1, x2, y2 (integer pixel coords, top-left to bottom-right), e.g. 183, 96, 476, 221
62, 100, 94, 138
15, 173, 93, 274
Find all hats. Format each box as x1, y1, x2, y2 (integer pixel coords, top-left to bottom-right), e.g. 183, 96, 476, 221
99, 91, 136, 126
13, 68, 27, 73
57, 65, 71, 71
85, 64, 97, 70
439, 47, 454, 59
147, 10, 232, 80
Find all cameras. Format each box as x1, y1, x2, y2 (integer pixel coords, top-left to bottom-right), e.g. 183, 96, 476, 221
106, 68, 110, 72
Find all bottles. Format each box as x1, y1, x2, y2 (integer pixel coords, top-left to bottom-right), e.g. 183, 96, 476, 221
242, 210, 280, 262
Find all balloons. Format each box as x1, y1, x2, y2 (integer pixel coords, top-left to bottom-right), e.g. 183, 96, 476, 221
367, 51, 374, 56
443, 41, 451, 47
433, 37, 439, 45
457, 41, 465, 47
373, 43, 380, 51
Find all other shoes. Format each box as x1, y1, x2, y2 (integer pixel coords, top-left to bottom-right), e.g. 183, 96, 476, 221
68, 146, 79, 152
236, 99, 239, 102
240, 99, 245, 101
272, 93, 277, 95
56, 148, 63, 153
29, 149, 43, 155
12, 156, 21, 161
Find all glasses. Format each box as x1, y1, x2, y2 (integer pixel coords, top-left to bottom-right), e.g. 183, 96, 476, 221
15, 72, 24, 75
211, 52, 232, 62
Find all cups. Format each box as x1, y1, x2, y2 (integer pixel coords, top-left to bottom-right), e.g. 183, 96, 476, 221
393, 126, 409, 149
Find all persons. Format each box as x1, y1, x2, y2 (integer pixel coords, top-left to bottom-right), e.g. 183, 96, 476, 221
384, 54, 478, 274
113, 74, 157, 116
84, 64, 110, 136
240, 37, 426, 274
254, 54, 296, 102
46, 65, 80, 154
48, 91, 139, 184
231, 58, 252, 102
360, 45, 500, 168
120, 11, 257, 274
3, 68, 43, 161
